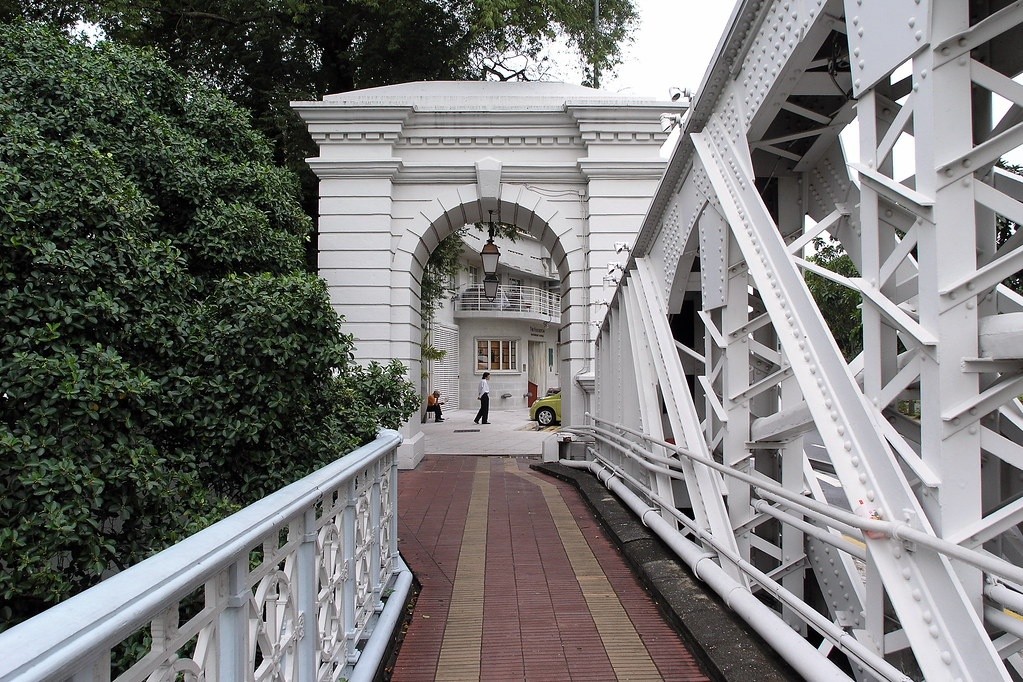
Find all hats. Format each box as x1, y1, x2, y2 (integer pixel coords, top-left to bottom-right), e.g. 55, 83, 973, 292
433, 391, 442, 395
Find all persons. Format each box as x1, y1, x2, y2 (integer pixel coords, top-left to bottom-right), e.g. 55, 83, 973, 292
474, 372, 491, 424
426, 391, 445, 423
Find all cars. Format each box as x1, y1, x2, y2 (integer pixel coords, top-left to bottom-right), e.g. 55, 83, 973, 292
530, 390, 562, 426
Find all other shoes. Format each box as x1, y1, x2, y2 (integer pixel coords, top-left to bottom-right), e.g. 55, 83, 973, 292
482, 422, 491, 424
438, 417, 445, 420
435, 419, 443, 422
474, 420, 479, 424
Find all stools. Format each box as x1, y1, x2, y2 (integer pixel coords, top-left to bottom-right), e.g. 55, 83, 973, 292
427, 411, 436, 423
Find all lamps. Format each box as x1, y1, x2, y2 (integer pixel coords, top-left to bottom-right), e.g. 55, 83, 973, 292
607, 263, 624, 274
480, 209, 501, 279
595, 299, 610, 313
483, 273, 500, 302
613, 242, 632, 254
603, 274, 620, 291
669, 86, 694, 101
590, 320, 600, 327
659, 112, 682, 133
